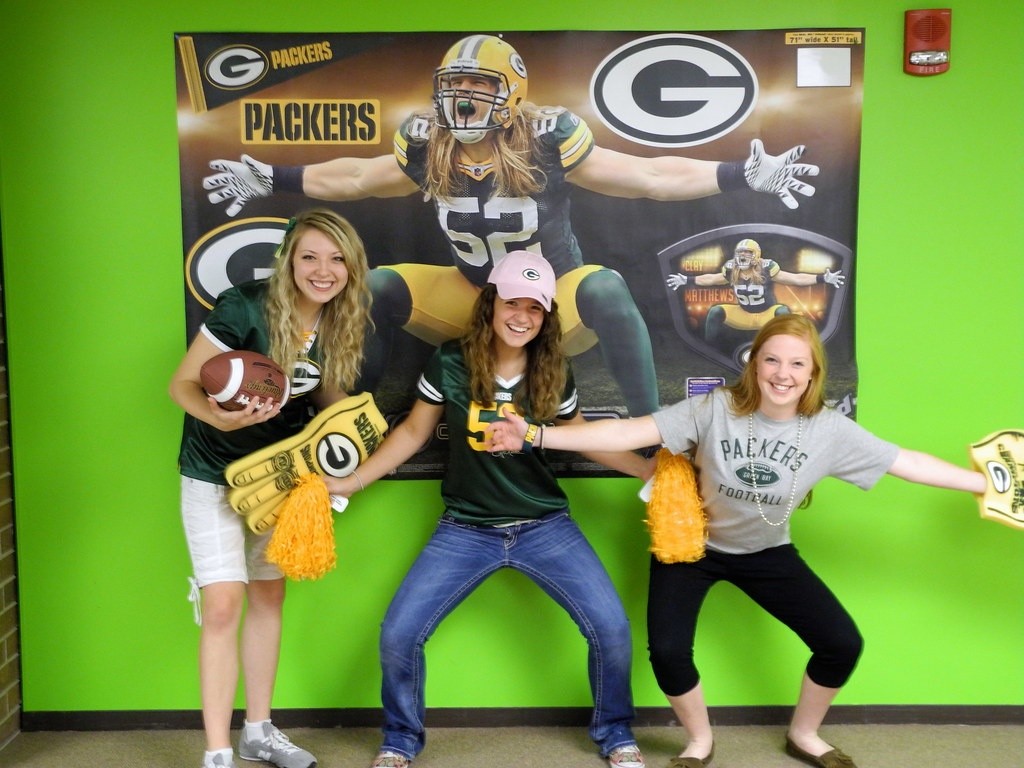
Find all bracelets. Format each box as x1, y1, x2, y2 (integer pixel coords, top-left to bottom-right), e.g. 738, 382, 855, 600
520, 423, 538, 454
352, 470, 367, 493
539, 424, 546, 450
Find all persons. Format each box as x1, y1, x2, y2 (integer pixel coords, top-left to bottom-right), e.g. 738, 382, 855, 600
198, 30, 819, 452
170, 208, 376, 767
329, 249, 664, 768
483, 308, 1023, 767
667, 237, 845, 348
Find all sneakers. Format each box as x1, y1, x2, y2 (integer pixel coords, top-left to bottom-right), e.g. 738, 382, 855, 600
238, 723, 317, 768
202, 753, 234, 768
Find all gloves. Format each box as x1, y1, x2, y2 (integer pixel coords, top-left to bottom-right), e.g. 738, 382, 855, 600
666, 273, 696, 291
816, 268, 845, 289
971, 430, 1024, 528
226, 392, 389, 537
717, 139, 818, 209
203, 153, 303, 218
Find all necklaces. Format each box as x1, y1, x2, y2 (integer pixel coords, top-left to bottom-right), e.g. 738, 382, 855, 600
749, 404, 803, 528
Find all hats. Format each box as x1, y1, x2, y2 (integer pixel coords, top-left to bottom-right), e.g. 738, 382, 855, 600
487, 250, 557, 312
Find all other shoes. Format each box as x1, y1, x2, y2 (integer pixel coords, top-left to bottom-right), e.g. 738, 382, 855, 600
610, 745, 645, 768
372, 752, 408, 768
786, 728, 857, 768
666, 741, 715, 768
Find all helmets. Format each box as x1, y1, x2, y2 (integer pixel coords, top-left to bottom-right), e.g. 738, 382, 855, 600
734, 239, 761, 269
431, 34, 527, 145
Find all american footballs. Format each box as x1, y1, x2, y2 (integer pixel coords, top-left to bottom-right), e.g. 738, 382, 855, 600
199, 349, 291, 412
